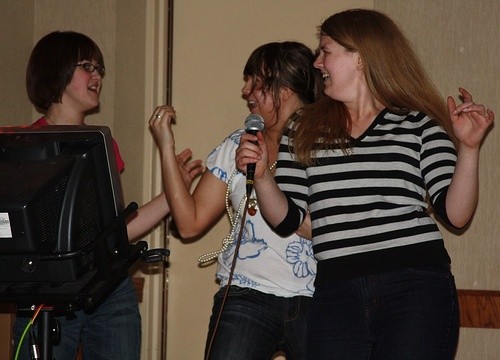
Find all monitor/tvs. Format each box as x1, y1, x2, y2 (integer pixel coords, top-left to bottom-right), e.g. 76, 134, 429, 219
0, 125, 146, 315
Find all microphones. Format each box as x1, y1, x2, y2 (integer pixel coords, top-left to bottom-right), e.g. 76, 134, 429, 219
244, 114, 265, 195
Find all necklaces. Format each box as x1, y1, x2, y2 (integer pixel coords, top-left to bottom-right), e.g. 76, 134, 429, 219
197, 160, 277, 262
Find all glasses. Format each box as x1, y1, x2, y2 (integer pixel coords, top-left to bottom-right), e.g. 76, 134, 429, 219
76, 63, 105, 78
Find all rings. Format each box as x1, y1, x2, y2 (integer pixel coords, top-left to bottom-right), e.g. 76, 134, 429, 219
156, 113, 160, 119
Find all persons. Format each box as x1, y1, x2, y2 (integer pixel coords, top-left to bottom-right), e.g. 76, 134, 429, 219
235, 8, 495, 360
12, 30, 204, 360
149, 41, 325, 360
